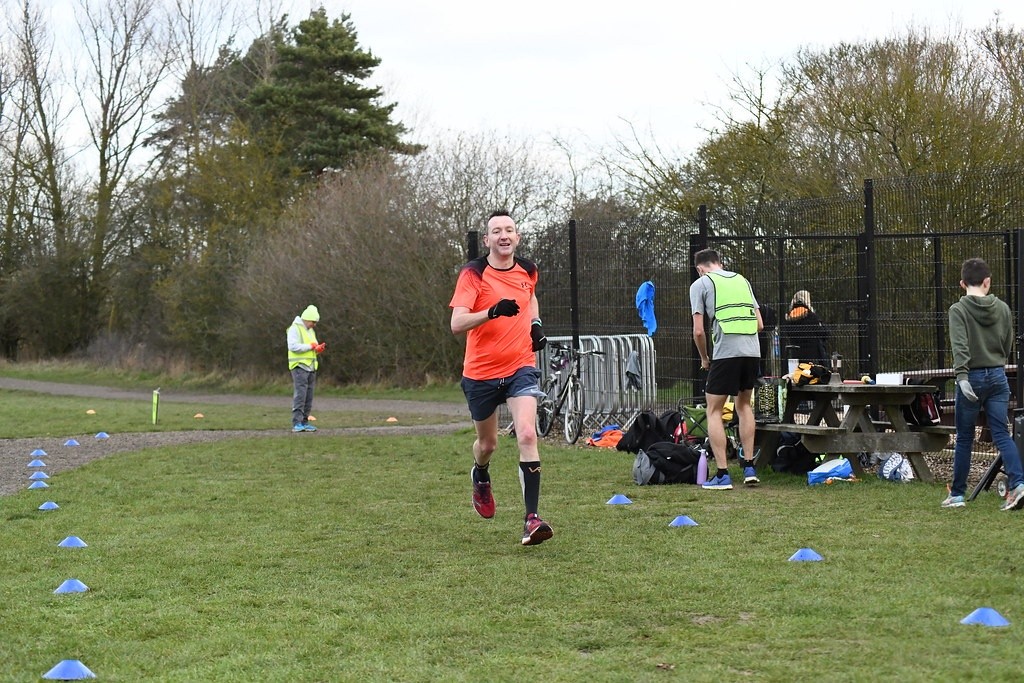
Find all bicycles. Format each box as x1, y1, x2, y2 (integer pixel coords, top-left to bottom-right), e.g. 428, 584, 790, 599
536, 341, 607, 446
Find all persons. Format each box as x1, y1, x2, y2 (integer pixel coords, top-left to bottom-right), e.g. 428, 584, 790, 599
448, 210, 554, 546
689, 248, 765, 490
286, 304, 326, 432
785, 290, 830, 369
941, 258, 1024, 510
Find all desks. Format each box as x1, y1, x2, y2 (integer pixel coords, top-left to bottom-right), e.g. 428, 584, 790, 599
782, 383, 939, 484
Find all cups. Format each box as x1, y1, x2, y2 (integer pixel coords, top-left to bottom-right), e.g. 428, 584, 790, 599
788, 359, 800, 384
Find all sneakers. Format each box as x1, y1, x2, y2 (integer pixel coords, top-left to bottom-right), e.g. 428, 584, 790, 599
292, 422, 305, 433
742, 466, 761, 485
470, 466, 496, 519
1000, 484, 1024, 512
702, 473, 733, 490
521, 513, 554, 546
941, 493, 966, 509
303, 422, 317, 432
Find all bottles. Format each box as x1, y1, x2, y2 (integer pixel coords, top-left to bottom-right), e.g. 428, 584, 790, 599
697, 449, 707, 485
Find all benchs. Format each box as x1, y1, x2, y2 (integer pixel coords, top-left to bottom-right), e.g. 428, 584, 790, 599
727, 420, 849, 436
868, 420, 956, 435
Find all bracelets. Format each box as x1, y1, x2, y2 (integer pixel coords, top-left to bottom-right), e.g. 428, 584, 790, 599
532, 321, 542, 327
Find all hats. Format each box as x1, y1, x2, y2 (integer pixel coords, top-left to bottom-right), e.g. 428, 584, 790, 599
300, 305, 320, 322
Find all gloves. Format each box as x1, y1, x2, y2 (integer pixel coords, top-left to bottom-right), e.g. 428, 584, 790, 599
312, 343, 325, 353
959, 380, 978, 403
529, 324, 547, 352
488, 299, 520, 320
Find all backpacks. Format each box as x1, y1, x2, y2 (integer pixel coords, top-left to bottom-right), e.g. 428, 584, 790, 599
616, 411, 666, 454
897, 375, 943, 427
632, 443, 710, 486
659, 410, 682, 441
